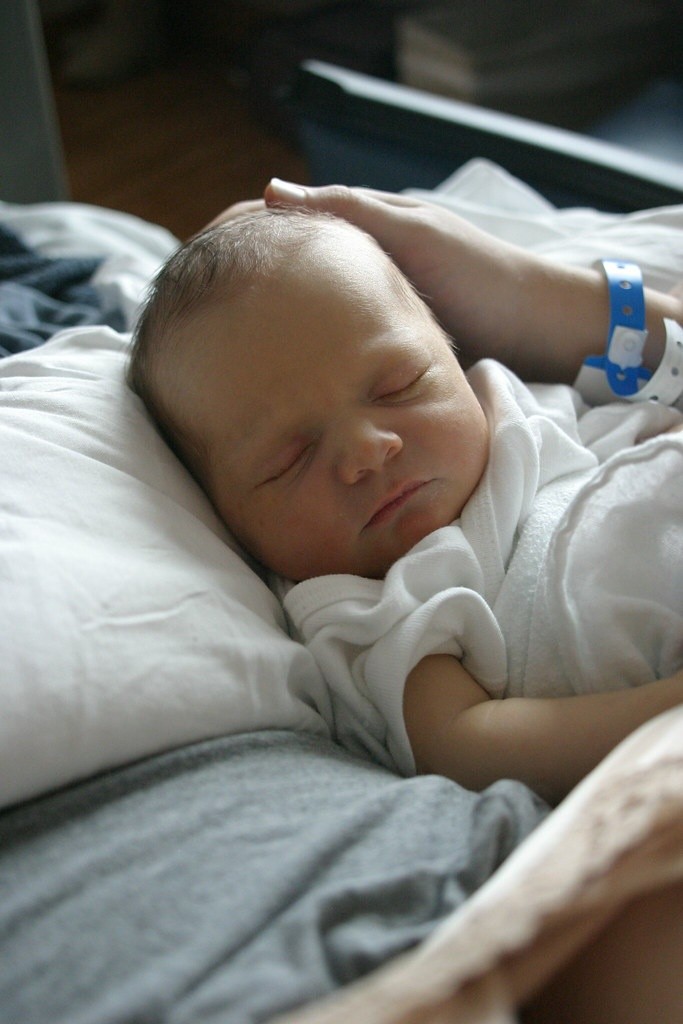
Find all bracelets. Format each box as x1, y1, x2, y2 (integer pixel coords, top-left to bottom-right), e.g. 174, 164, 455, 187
575, 254, 683, 408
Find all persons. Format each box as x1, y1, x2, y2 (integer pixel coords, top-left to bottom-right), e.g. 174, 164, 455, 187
125, 176, 683, 1023
